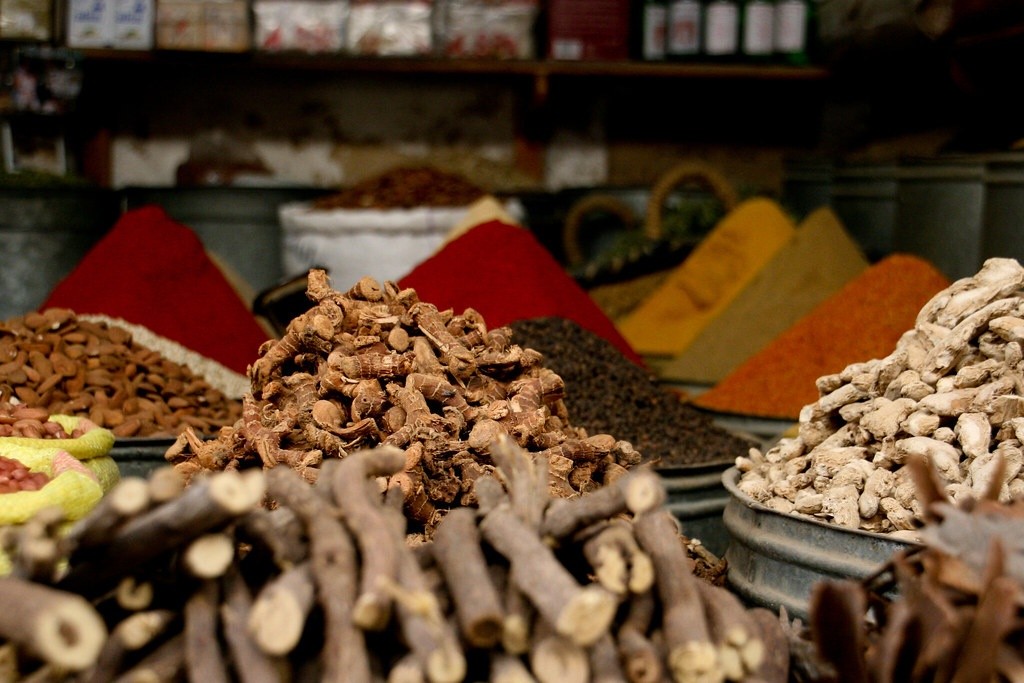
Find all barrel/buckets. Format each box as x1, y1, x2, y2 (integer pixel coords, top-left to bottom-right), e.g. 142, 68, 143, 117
108, 381, 915, 631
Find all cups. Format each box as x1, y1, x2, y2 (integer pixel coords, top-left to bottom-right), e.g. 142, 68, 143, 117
706, 2, 738, 55
777, 0, 807, 55
670, 0, 698, 53
644, 5, 664, 59
745, 2, 774, 57
